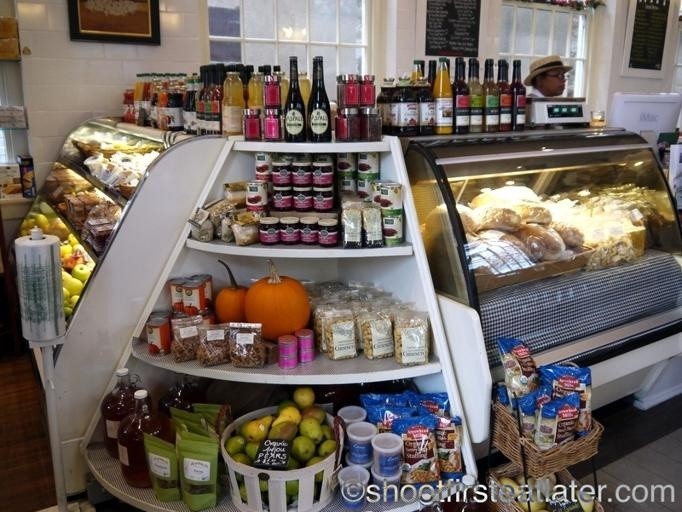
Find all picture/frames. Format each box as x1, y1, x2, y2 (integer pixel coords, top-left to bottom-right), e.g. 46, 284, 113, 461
68, 0, 160, 45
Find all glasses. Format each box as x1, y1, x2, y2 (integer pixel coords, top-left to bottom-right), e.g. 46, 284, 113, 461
547, 72, 567, 81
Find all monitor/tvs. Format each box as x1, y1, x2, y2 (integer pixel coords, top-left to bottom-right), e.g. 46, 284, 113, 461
606, 91, 682, 168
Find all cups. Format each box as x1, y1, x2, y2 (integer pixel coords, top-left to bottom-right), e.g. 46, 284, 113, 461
591, 110, 606, 127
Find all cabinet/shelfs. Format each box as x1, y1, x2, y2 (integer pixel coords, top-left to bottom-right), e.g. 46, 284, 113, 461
0, 117, 682, 512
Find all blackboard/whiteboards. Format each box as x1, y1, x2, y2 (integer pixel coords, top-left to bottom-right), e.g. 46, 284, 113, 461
415, 0, 489, 61
620, 0, 675, 80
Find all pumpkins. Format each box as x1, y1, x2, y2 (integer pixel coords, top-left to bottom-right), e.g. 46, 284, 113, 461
214, 258, 249, 322
244, 257, 309, 342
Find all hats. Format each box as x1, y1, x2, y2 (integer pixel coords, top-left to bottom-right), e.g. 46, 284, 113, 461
524, 56, 573, 86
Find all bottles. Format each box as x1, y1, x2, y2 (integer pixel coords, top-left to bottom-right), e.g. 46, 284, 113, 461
295, 329, 315, 363
334, 74, 383, 141
374, 73, 435, 137
283, 54, 306, 143
304, 54, 331, 143
297, 71, 311, 101
195, 63, 289, 138
102, 367, 203, 493
278, 334, 298, 369
122, 71, 200, 132
415, 55, 528, 134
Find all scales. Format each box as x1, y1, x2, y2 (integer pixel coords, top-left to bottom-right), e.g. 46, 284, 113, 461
525, 97, 591, 130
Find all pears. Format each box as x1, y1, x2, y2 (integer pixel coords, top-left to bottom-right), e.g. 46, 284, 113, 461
227, 388, 337, 501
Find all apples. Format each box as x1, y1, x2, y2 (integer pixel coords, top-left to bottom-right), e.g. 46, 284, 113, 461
20, 213, 91, 318
498, 472, 594, 512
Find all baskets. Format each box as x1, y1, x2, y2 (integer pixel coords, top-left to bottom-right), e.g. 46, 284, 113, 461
221, 403, 345, 512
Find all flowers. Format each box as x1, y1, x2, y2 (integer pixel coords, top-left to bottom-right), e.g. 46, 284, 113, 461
550, 0, 605, 11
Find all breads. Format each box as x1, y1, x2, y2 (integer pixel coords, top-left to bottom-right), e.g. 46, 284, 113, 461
40, 135, 160, 250
423, 185, 589, 277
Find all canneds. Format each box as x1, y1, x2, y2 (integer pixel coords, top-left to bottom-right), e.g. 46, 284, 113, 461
247, 153, 402, 247
